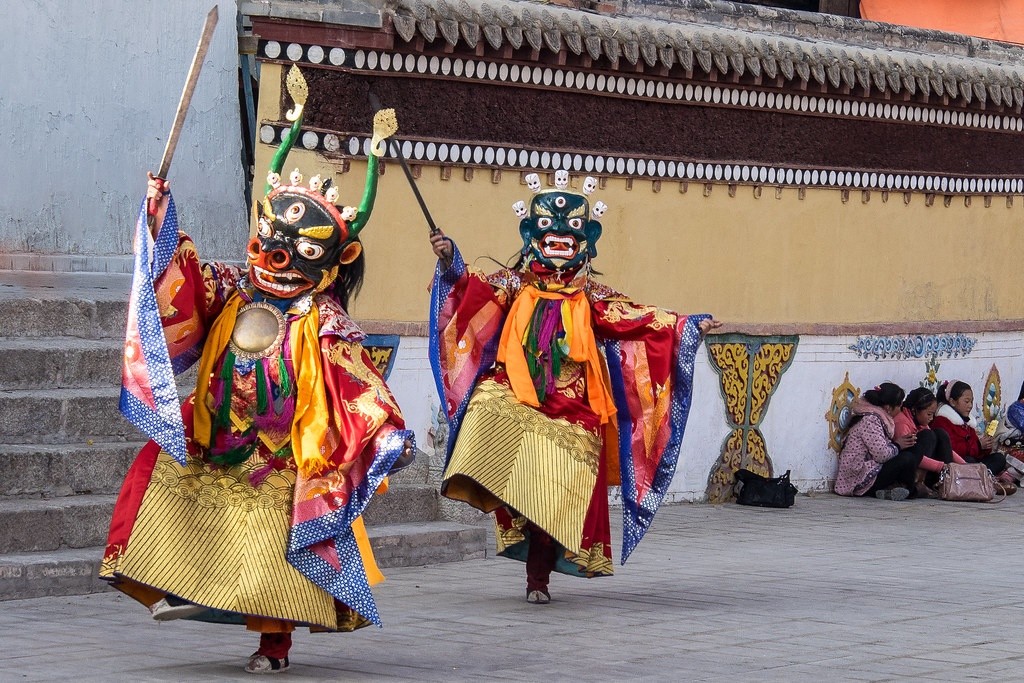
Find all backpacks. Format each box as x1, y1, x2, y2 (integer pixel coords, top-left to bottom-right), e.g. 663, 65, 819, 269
734, 469, 798, 508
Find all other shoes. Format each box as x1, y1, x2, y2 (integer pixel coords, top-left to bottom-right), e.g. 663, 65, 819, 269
245, 655, 290, 674
876, 488, 909, 500
527, 588, 551, 604
152, 594, 210, 620
915, 481, 938, 499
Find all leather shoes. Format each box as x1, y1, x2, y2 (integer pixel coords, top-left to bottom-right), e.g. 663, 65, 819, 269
994, 482, 1017, 495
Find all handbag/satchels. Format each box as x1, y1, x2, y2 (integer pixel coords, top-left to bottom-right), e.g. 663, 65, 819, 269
932, 462, 1006, 503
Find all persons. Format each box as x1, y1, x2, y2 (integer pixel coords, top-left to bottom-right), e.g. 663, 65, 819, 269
835, 384, 923, 501
430, 167, 725, 602
889, 386, 967, 500
985, 376, 1024, 491
101, 164, 417, 675
930, 380, 1018, 497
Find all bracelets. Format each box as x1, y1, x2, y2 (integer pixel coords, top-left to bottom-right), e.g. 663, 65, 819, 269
893, 443, 901, 452
